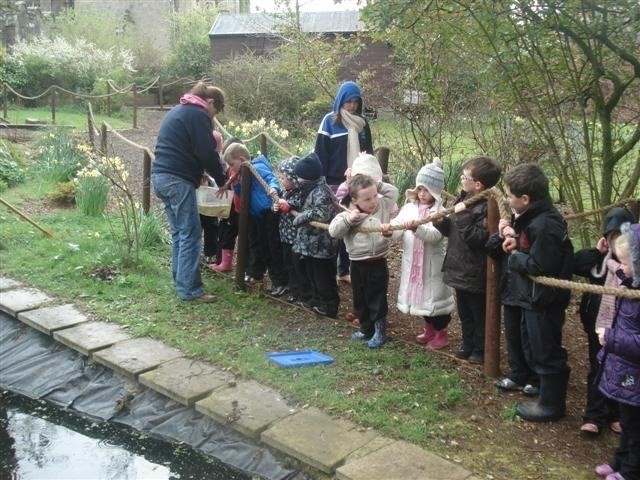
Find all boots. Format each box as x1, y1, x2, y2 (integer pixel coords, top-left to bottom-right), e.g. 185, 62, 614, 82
205, 248, 233, 272
516, 365, 571, 421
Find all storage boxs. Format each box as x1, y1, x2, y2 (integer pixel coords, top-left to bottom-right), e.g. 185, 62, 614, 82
195, 186, 234, 219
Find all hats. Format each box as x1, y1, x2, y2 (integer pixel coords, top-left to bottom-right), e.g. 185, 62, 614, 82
351, 151, 383, 185
405, 157, 445, 206
294, 153, 321, 180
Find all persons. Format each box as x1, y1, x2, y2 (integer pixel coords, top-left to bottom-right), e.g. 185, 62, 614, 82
568, 206, 639, 436
290, 152, 343, 318
327, 173, 401, 349
332, 149, 400, 327
378, 155, 456, 350
224, 142, 281, 285
482, 211, 543, 396
427, 156, 503, 365
592, 222, 639, 480
151, 82, 233, 304
208, 137, 246, 273
196, 129, 222, 264
311, 82, 376, 285
501, 161, 576, 423
270, 156, 313, 304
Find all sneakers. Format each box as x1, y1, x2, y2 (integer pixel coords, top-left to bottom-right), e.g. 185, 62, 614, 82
198, 295, 218, 302
494, 378, 539, 395
595, 465, 624, 480
232, 274, 263, 283
267, 285, 336, 317
581, 423, 599, 433
345, 312, 386, 348
416, 323, 449, 350
456, 344, 484, 364
609, 421, 623, 433
